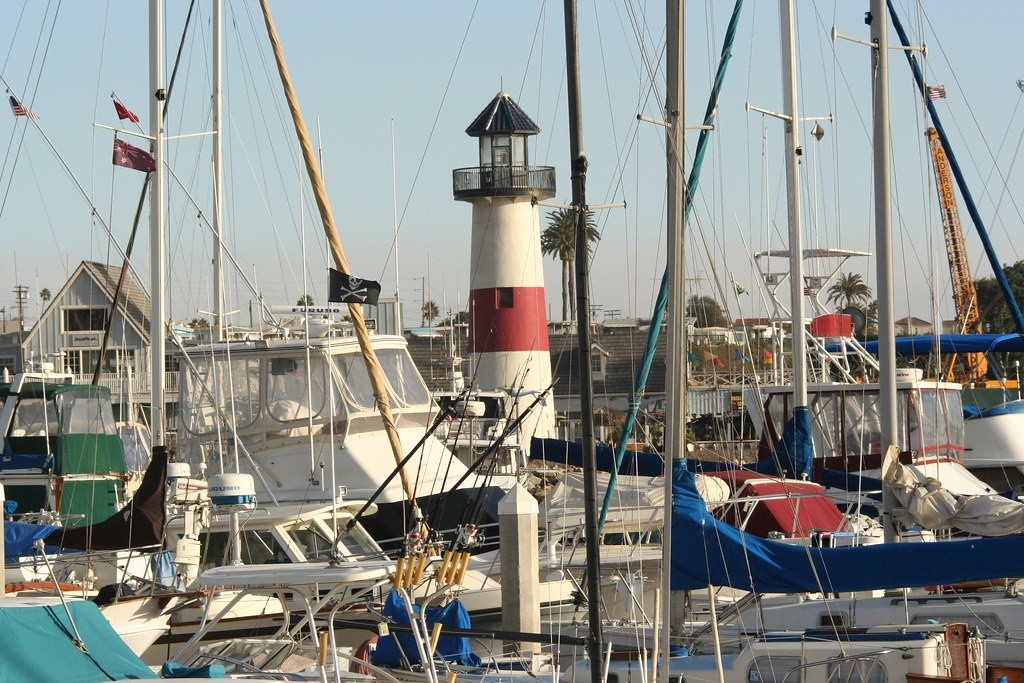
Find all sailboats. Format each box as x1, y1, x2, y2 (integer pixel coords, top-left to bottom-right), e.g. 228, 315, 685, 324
0, 0, 1024, 683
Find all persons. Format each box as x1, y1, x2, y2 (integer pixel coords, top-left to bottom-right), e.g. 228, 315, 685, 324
595, 435, 643, 452
835, 370, 872, 455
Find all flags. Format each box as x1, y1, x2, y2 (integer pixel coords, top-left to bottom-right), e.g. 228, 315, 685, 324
328, 268, 381, 306
9, 96, 40, 119
1017, 80, 1024, 85
112, 137, 156, 174
927, 85, 946, 100
115, 102, 139, 122
